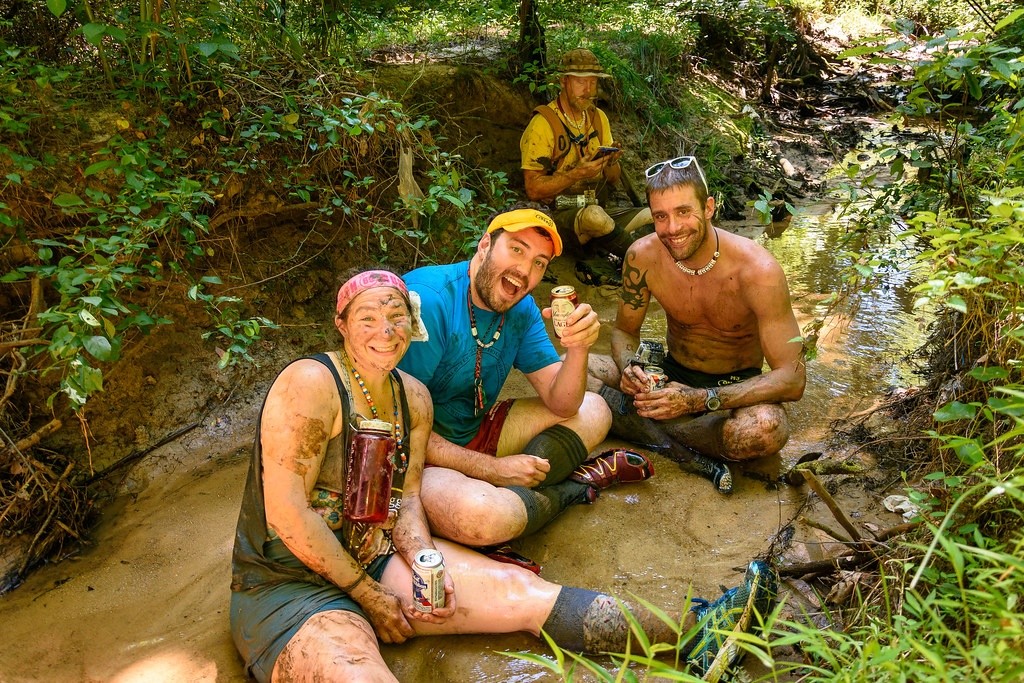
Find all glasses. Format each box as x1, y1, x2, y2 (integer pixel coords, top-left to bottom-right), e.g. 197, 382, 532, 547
645, 156, 710, 195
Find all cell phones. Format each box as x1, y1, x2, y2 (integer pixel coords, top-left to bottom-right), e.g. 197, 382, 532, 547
590, 148, 618, 162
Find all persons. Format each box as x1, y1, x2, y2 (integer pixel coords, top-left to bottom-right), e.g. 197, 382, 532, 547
393, 209, 657, 547
231, 270, 777, 683
518, 50, 659, 286
559, 156, 807, 496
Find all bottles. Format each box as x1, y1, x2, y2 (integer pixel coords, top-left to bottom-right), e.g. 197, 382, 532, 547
344, 420, 396, 523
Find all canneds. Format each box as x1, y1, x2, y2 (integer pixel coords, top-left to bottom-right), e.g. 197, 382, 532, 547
549, 285, 579, 339
411, 548, 445, 614
642, 366, 664, 410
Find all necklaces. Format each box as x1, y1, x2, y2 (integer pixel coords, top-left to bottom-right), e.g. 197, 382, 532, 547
675, 228, 720, 276
556, 95, 586, 129
341, 348, 407, 474
467, 283, 506, 349
467, 297, 502, 417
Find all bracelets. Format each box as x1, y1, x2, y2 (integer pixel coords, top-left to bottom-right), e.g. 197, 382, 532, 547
341, 569, 367, 595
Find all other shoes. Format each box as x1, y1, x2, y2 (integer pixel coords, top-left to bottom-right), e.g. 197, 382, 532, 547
567, 447, 654, 506
680, 453, 732, 495
480, 542, 542, 579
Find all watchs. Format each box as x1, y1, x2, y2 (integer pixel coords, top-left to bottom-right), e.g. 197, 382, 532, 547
705, 388, 722, 412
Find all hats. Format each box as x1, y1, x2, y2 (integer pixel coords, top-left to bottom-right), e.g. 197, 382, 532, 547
486, 209, 563, 256
554, 47, 612, 79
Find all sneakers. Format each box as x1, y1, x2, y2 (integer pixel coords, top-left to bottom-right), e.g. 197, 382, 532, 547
675, 560, 777, 682
